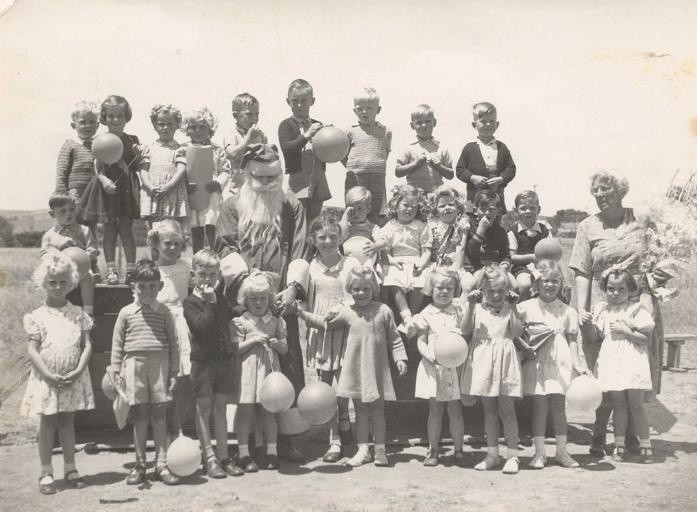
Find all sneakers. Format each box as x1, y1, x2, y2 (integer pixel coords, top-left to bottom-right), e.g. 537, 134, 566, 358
346, 448, 373, 467
529, 453, 548, 469
474, 454, 502, 472
501, 456, 520, 474
423, 448, 441, 466
554, 452, 580, 469
373, 449, 390, 466
249, 437, 313, 471
453, 449, 472, 467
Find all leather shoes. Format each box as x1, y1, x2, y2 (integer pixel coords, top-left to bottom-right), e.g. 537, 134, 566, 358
237, 455, 259, 473
90, 269, 136, 286
220, 458, 244, 476
65, 469, 87, 489
155, 464, 181, 485
205, 460, 228, 479
126, 464, 147, 486
38, 473, 57, 495
338, 414, 353, 445
322, 443, 344, 463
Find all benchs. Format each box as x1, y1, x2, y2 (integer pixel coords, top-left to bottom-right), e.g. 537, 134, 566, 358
657, 333, 696, 375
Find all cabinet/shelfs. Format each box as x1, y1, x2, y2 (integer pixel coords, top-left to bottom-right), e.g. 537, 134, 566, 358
67, 283, 135, 431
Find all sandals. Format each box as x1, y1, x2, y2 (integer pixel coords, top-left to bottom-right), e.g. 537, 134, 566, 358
611, 445, 629, 463
638, 446, 656, 464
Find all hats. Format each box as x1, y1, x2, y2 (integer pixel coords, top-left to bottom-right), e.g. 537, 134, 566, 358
101, 365, 121, 401
238, 140, 283, 178
